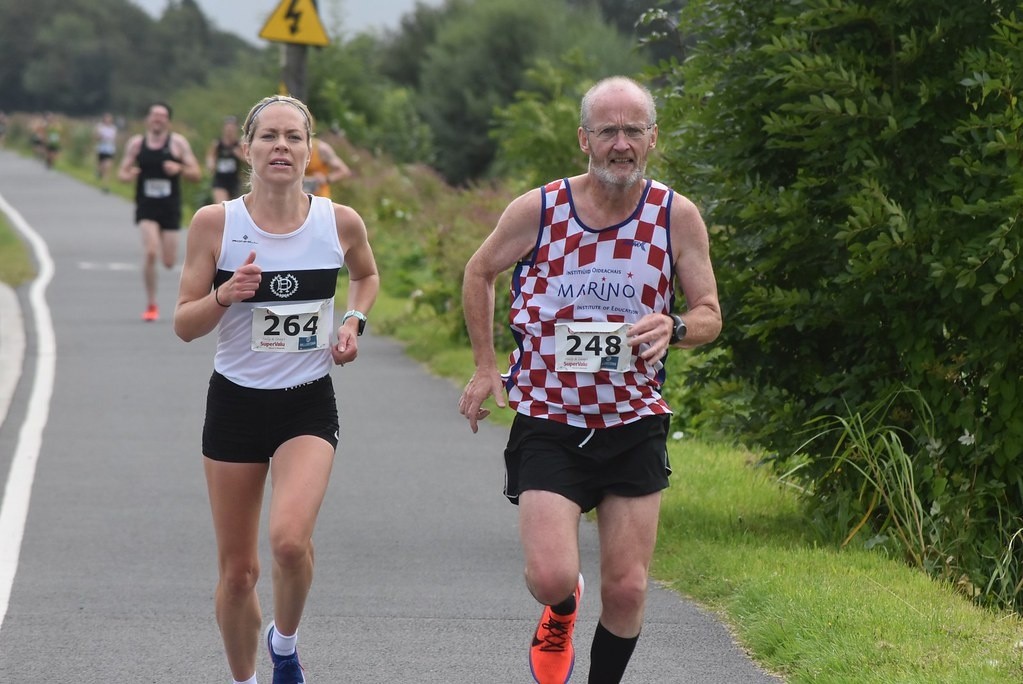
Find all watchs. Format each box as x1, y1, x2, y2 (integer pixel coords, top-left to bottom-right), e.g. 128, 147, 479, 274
668, 314, 687, 345
342, 310, 367, 336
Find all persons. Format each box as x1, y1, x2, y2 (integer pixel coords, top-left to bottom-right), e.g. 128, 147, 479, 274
30, 111, 65, 171
206, 114, 247, 204
302, 137, 352, 199
118, 102, 203, 320
91, 113, 118, 196
172, 95, 380, 684
0, 110, 8, 142
458, 75, 722, 684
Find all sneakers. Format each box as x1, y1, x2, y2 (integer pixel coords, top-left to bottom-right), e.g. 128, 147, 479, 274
264, 620, 307, 684
529, 575, 585, 684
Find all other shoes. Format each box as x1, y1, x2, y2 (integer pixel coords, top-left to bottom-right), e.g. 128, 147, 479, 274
144, 304, 158, 321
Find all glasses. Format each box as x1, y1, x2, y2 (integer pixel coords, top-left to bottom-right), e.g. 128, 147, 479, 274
584, 125, 653, 141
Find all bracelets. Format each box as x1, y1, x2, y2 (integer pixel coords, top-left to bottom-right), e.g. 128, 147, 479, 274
216, 288, 231, 307
325, 176, 331, 184
179, 166, 184, 173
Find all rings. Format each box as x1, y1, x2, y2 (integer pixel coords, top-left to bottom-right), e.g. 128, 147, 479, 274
341, 362, 343, 367
